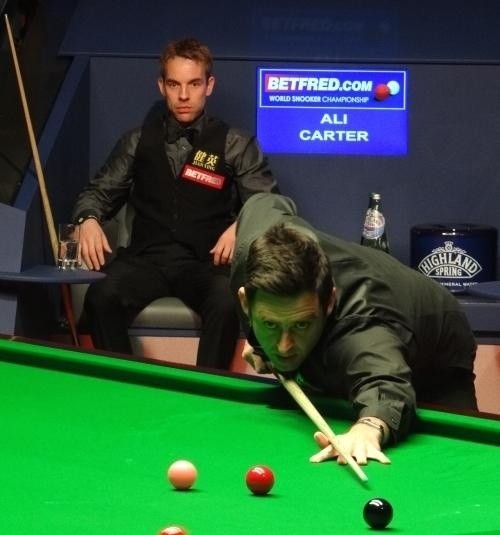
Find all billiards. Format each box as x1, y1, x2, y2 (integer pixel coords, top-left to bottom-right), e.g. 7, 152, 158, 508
246, 466, 274, 494
166, 460, 198, 491
159, 526, 186, 535
363, 498, 392, 529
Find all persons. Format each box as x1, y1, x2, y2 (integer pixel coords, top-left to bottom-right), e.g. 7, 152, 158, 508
72, 39, 281, 369
232, 192, 478, 465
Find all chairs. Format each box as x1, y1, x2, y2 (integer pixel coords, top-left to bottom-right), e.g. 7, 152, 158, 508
69, 201, 274, 380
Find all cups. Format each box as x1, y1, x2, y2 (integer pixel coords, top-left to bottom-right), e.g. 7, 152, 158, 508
56, 222, 81, 272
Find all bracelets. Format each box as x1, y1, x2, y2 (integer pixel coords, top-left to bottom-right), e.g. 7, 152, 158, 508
357, 419, 384, 445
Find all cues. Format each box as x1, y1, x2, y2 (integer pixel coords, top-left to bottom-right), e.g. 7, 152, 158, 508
4, 13, 80, 346
274, 371, 368, 484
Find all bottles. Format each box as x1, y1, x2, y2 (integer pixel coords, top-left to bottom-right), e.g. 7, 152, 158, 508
360, 190, 391, 255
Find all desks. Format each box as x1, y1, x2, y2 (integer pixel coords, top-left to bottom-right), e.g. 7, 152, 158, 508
0, 330, 500, 534
